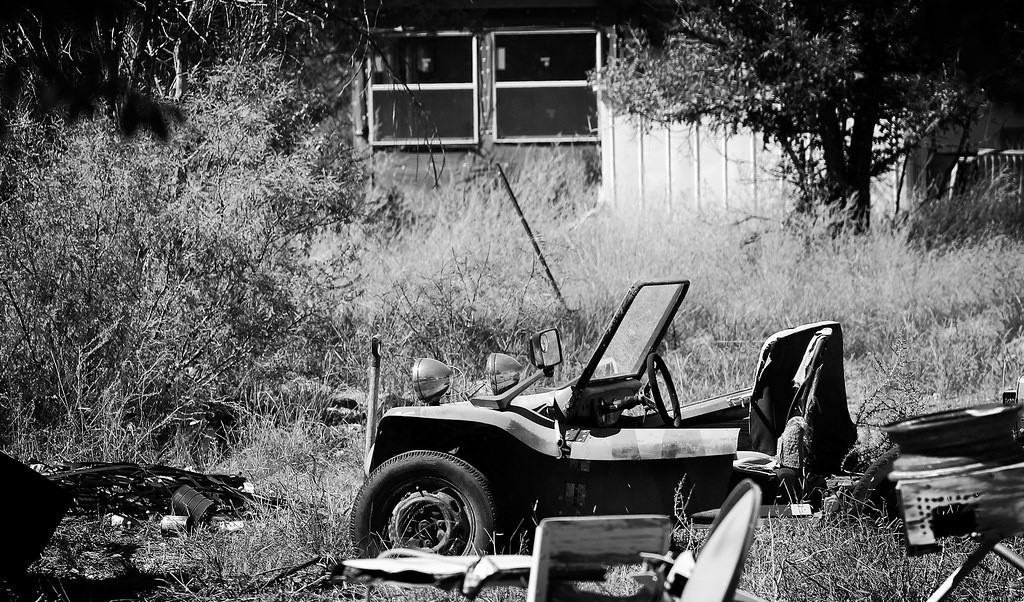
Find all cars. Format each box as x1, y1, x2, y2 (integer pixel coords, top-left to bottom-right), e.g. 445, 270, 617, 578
350, 280, 858, 558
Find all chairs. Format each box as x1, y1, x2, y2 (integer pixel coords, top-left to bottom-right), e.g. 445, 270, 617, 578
748, 320, 858, 475
733, 328, 834, 506
